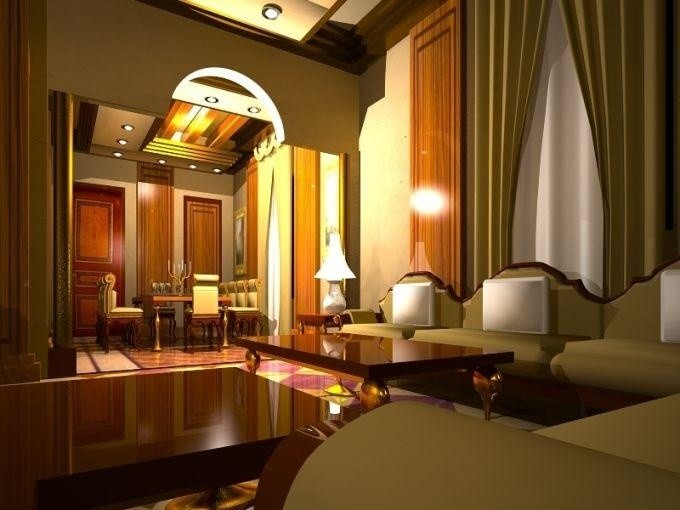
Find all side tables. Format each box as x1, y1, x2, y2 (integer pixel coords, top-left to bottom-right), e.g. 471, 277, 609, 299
298, 312, 383, 335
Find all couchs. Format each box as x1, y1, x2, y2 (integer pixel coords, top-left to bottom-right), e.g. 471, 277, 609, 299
257, 261, 680, 510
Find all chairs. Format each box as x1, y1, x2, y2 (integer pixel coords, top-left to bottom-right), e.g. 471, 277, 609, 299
97, 274, 263, 353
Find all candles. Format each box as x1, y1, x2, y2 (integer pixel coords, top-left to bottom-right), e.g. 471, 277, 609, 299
167, 259, 192, 273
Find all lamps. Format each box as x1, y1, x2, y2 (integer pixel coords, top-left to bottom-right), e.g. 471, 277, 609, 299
314, 234, 357, 314
